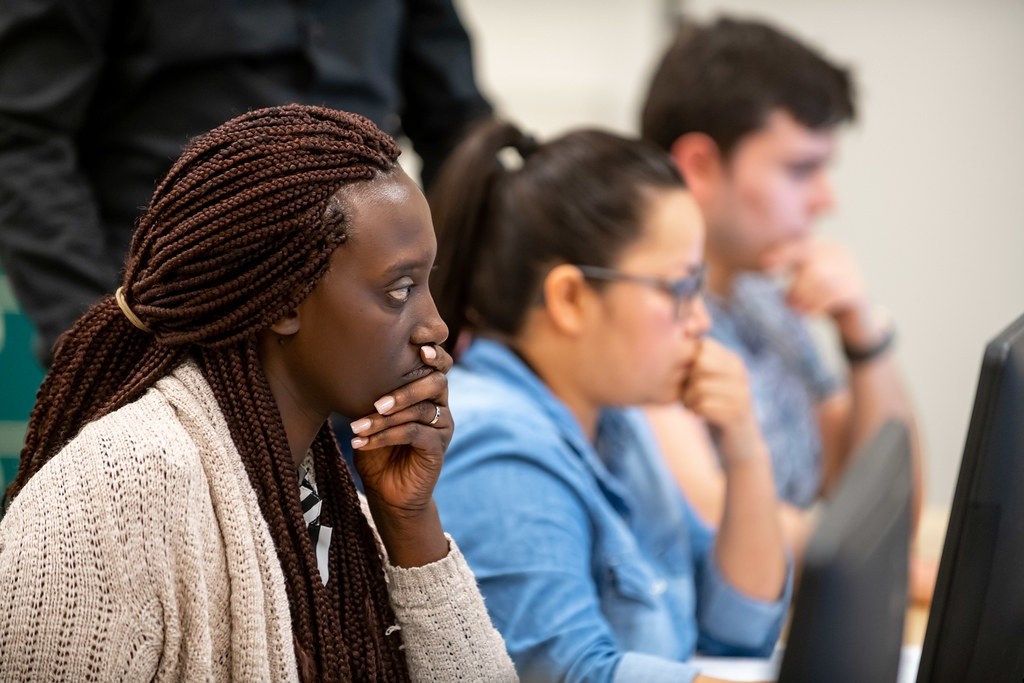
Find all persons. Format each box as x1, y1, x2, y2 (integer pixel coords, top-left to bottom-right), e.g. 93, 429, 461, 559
1, 0, 493, 371
0, 104, 520, 682
637, 16, 922, 599
346, 118, 792, 683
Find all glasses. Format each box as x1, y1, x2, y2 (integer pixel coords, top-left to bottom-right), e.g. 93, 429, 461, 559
574, 262, 705, 307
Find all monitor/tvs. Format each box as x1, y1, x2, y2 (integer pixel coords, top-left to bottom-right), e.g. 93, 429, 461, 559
915, 315, 1024, 683
773, 417, 912, 683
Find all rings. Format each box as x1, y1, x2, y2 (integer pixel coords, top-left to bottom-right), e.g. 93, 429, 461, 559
428, 404, 440, 426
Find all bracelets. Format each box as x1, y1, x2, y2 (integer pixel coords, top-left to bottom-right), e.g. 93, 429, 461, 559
842, 320, 892, 363
